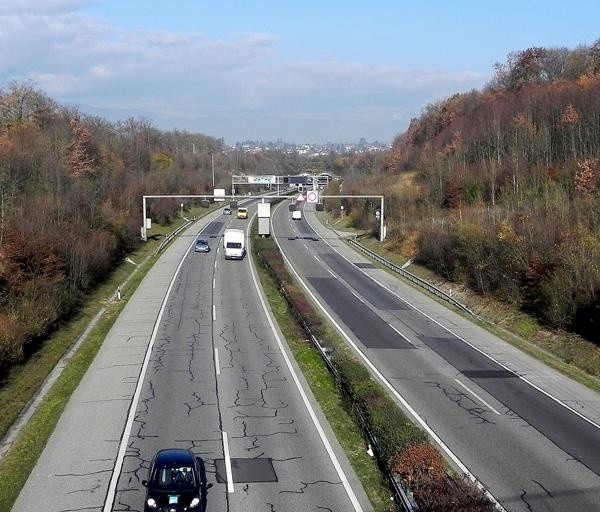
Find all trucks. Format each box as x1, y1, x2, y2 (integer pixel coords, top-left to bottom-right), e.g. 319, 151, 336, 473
237, 208, 248, 219
223, 229, 246, 260
230, 200, 238, 208
292, 210, 302, 220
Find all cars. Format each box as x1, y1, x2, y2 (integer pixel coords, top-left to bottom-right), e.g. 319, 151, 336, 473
195, 239, 209, 252
142, 449, 213, 512
223, 208, 231, 215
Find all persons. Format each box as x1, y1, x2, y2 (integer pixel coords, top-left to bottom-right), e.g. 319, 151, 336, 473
174, 472, 193, 483
